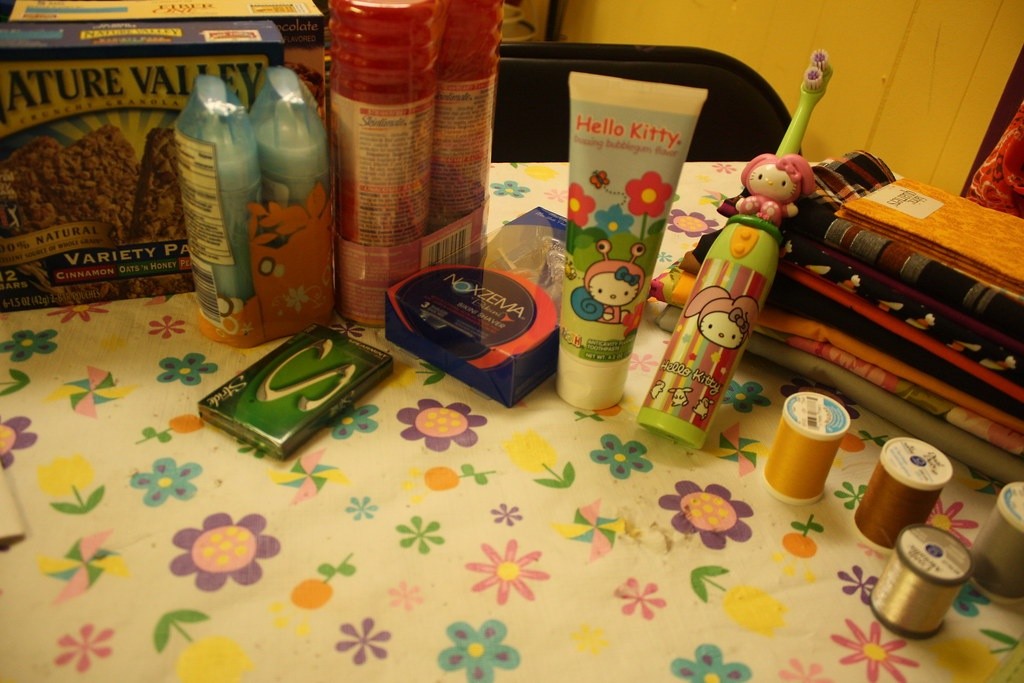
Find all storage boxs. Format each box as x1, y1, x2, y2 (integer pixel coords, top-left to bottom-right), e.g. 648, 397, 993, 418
0, 0, 336, 311
196, 323, 394, 461
386, 206, 567, 409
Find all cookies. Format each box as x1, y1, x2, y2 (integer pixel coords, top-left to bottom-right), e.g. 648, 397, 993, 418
0, 122, 197, 303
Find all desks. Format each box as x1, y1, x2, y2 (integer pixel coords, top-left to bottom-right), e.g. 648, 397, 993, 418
0, 157, 1024, 683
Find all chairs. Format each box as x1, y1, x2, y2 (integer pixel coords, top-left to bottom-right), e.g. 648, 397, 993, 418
490, 43, 802, 163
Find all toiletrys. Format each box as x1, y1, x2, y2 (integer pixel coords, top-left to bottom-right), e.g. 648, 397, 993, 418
556, 71, 708, 411
175, 0, 504, 349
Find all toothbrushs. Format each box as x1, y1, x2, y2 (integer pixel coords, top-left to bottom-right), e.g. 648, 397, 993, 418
638, 48, 833, 449
